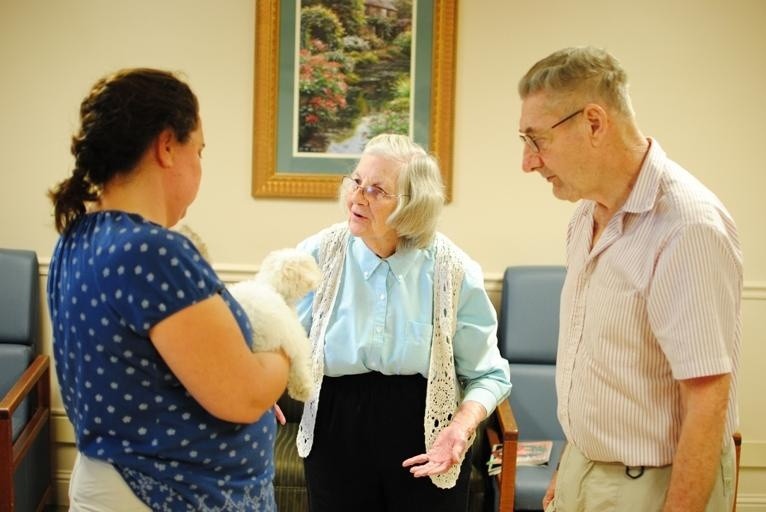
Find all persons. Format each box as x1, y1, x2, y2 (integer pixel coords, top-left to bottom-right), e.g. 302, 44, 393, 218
269, 130, 518, 512
44, 63, 293, 512
511, 39, 750, 512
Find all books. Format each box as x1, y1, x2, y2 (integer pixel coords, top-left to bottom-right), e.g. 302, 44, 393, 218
485, 434, 557, 479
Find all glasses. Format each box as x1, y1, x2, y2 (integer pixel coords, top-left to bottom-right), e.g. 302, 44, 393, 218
339, 176, 409, 203
516, 109, 582, 153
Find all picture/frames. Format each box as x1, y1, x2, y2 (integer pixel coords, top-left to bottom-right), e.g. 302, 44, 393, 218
248, 0, 458, 207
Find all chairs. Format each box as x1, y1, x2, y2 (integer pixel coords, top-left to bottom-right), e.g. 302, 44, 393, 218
486, 265, 745, 511
0, 247, 59, 511
